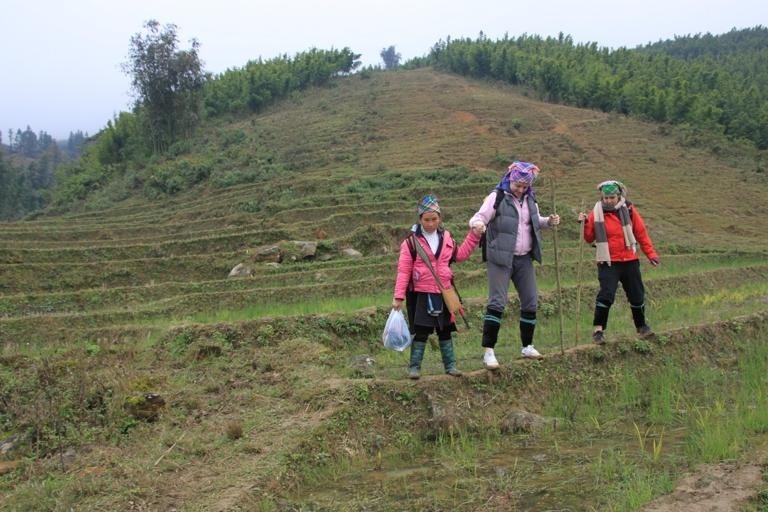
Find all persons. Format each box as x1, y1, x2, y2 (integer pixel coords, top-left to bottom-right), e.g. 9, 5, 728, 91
468, 160, 560, 370
392, 193, 485, 379
576, 180, 660, 344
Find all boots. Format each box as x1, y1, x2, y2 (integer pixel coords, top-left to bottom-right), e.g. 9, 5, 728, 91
409, 340, 425, 380
439, 339, 461, 377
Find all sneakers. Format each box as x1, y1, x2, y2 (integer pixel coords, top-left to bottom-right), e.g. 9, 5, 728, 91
522, 345, 542, 359
636, 325, 654, 338
483, 351, 500, 370
592, 331, 605, 345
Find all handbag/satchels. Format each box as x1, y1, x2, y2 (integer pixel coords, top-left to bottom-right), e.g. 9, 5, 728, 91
441, 287, 461, 315
